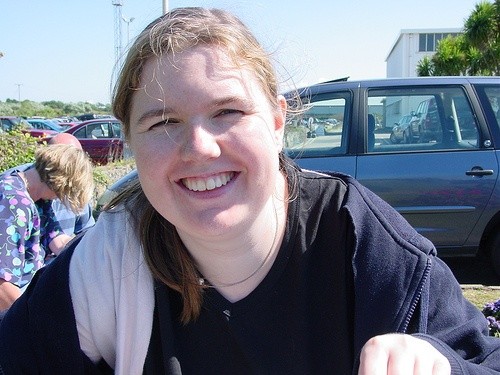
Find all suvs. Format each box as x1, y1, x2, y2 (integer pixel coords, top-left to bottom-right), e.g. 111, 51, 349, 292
92, 77, 500, 284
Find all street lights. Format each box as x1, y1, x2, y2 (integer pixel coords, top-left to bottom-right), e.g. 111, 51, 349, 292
121, 15, 135, 53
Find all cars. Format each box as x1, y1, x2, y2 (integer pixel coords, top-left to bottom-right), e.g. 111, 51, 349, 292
1, 114, 124, 166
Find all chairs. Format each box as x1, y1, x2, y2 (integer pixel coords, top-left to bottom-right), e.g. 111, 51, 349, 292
368, 114, 375, 151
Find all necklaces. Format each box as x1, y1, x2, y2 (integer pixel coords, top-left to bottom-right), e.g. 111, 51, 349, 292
198, 197, 278, 287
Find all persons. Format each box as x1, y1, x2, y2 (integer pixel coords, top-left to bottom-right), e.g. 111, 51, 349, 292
0, 7, 500, 375
0, 133, 96, 322
